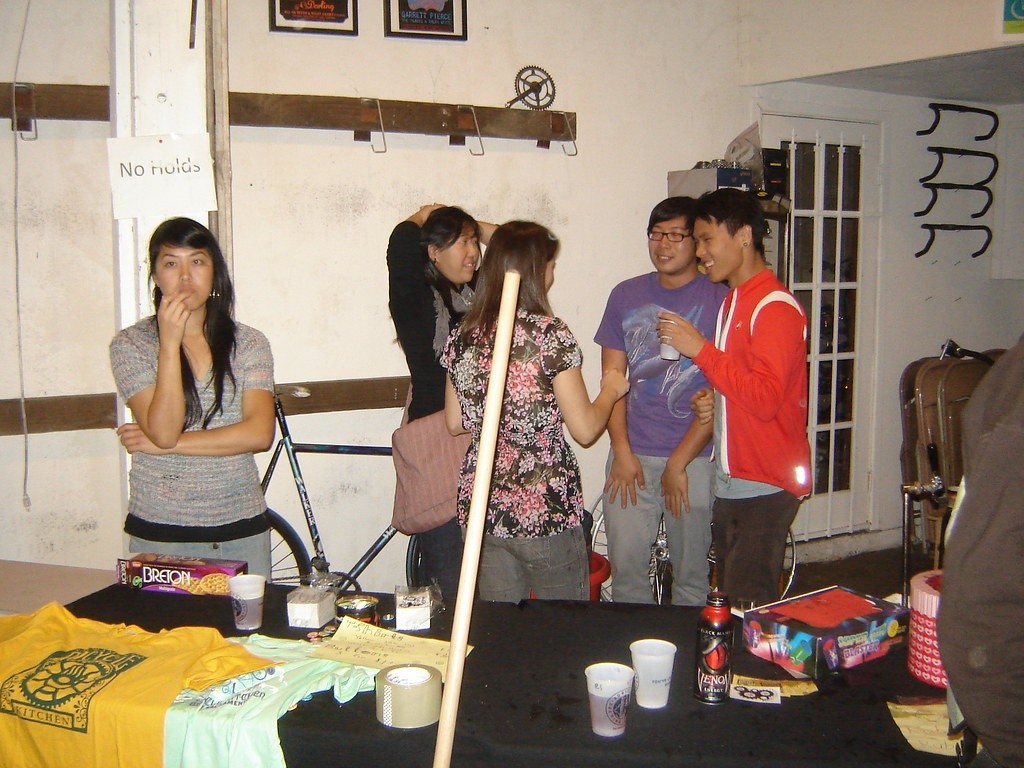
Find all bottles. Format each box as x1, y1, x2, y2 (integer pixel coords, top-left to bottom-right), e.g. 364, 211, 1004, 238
693, 592, 735, 706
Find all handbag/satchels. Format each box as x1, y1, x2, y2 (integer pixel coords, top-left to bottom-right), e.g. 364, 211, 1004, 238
390, 408, 471, 535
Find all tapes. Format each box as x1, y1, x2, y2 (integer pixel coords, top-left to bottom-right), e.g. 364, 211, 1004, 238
374, 662, 443, 730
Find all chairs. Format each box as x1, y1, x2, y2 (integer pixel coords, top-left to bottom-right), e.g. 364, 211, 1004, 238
898, 350, 1008, 611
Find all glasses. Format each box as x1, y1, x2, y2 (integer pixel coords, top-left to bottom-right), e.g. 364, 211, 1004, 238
647, 230, 691, 242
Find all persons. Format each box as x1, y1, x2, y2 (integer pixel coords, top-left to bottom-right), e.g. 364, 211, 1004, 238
110, 217, 275, 583
656, 188, 811, 610
936, 332, 1024, 768
386, 204, 501, 599
439, 221, 630, 605
594, 196, 730, 607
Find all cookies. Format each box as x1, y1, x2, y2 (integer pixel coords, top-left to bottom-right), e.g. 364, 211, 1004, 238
172, 572, 235, 596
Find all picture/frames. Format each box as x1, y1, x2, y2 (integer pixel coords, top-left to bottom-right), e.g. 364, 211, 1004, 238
383, 0, 468, 41
268, 0, 359, 37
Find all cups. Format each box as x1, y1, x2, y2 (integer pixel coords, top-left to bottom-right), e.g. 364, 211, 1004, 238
334, 594, 384, 630
228, 574, 266, 631
658, 317, 683, 361
584, 662, 634, 737
629, 638, 677, 709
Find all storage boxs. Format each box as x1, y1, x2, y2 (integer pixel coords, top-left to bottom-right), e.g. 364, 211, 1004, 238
115, 551, 248, 596
741, 584, 910, 680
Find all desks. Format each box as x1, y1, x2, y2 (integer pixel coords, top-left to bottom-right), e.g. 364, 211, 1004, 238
0, 557, 992, 768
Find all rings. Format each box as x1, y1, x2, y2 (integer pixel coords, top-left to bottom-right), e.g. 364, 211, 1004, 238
161, 298, 170, 306
667, 338, 670, 345
